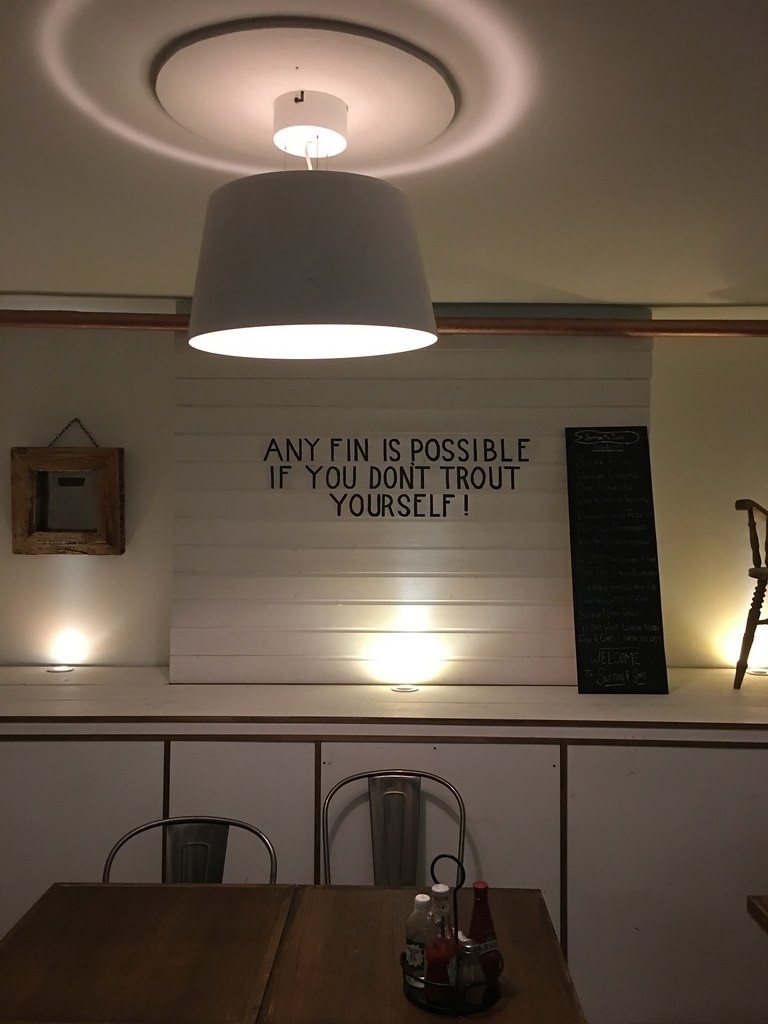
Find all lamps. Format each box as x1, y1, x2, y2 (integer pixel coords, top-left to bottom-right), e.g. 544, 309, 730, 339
188, 90, 438, 359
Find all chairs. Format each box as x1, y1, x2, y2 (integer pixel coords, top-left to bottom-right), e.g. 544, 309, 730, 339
102, 815, 277, 882
323, 769, 466, 886
733, 499, 768, 688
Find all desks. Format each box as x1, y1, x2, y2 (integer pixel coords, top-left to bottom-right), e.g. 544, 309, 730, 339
1, 881, 588, 1024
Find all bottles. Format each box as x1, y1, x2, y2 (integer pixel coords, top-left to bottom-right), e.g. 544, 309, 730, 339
468, 880, 500, 983
423, 883, 459, 1007
405, 893, 432, 988
